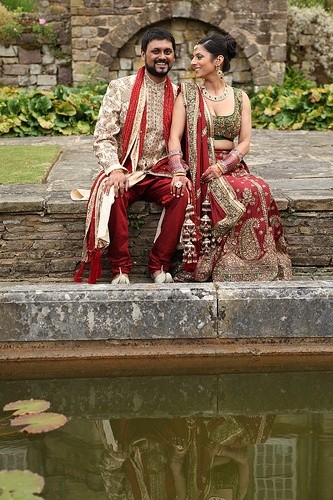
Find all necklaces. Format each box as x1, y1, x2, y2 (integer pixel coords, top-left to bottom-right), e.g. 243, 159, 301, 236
200, 82, 229, 102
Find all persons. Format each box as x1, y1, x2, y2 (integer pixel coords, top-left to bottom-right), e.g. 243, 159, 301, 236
82, 30, 188, 284
168, 36, 293, 282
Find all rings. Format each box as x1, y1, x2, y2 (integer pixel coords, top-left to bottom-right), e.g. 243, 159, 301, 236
175, 180, 184, 189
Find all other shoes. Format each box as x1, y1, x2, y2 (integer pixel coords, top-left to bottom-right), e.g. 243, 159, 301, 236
151, 265, 174, 283
111, 267, 130, 284
